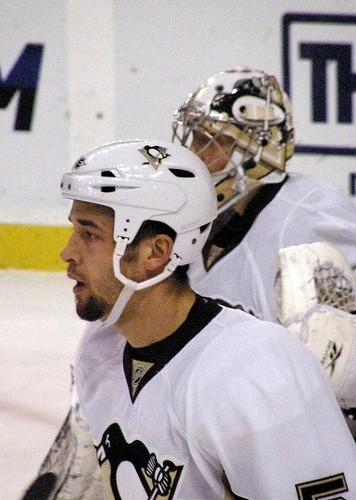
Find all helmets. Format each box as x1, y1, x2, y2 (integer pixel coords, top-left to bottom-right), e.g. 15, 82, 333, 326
60, 141, 217, 269
172, 70, 293, 213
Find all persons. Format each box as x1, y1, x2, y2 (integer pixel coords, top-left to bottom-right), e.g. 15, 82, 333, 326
171, 67, 356, 442
60, 140, 356, 500
